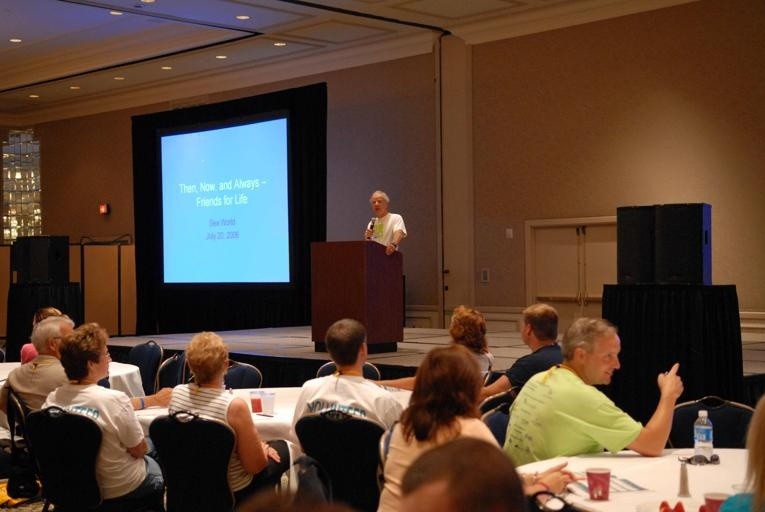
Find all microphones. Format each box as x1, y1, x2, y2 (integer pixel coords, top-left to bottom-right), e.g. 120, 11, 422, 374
367, 218, 376, 240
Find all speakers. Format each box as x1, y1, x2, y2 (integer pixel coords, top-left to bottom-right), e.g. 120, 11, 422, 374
16, 235, 70, 284
617, 204, 712, 287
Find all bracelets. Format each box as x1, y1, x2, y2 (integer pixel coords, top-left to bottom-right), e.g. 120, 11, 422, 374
389, 241, 398, 249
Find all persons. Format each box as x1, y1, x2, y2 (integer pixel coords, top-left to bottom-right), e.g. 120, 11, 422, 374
503, 316, 683, 469
718, 394, 764, 511
244, 486, 343, 511
0, 304, 162, 502
287, 306, 493, 472
165, 329, 294, 504
363, 190, 408, 259
380, 304, 585, 511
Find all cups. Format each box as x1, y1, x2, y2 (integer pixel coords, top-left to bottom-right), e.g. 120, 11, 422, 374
250, 391, 276, 417
732, 485, 758, 494
586, 467, 610, 500
703, 491, 732, 511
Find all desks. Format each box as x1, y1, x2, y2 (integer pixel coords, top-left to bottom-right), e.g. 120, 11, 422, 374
2, 280, 86, 363
593, 282, 749, 435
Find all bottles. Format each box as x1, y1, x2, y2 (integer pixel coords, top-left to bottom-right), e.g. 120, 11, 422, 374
693, 410, 714, 460
676, 456, 692, 498
